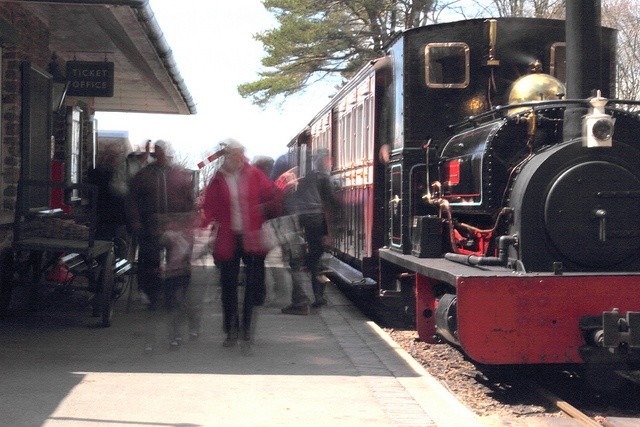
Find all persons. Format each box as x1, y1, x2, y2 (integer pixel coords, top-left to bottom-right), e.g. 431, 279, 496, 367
87, 139, 207, 346
201, 130, 344, 348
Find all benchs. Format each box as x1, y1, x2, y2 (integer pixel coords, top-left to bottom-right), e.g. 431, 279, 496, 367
51, 238, 132, 311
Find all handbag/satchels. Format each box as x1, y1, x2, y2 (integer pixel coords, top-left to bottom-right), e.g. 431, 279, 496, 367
262, 215, 296, 247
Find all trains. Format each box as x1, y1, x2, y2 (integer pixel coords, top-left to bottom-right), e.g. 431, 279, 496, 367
286, 0, 640, 396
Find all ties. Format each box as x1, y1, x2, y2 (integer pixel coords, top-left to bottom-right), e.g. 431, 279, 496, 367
159, 169, 166, 213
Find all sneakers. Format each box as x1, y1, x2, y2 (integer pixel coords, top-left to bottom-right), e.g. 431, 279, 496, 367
282, 303, 309, 314
170, 338, 182, 351
150, 296, 163, 311
312, 299, 327, 307
242, 330, 252, 341
222, 330, 237, 348
189, 329, 198, 338
164, 296, 178, 309
